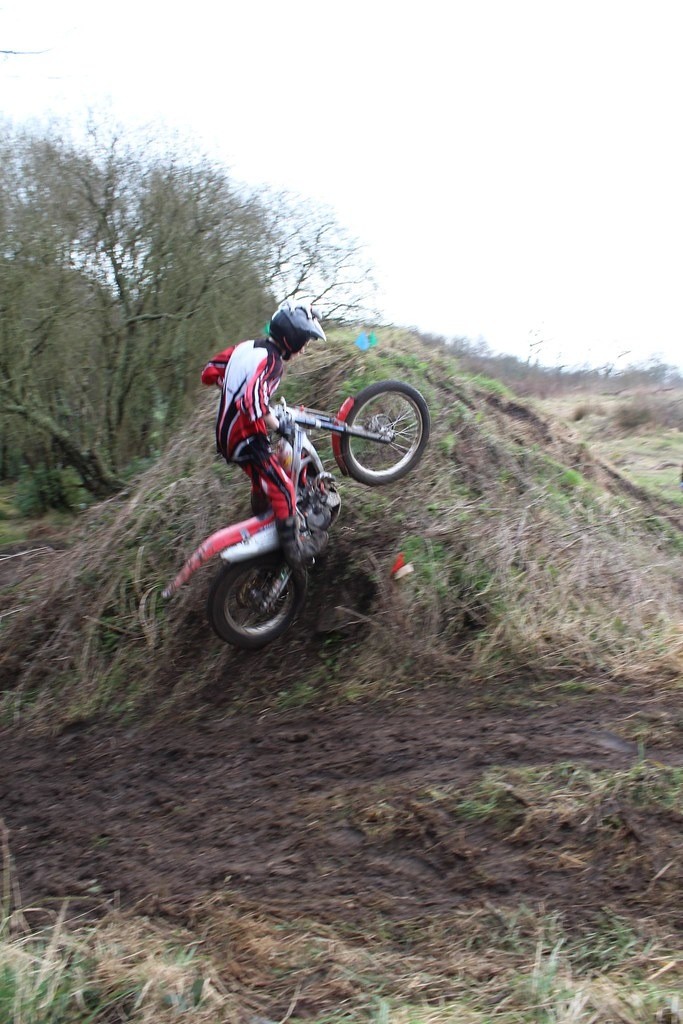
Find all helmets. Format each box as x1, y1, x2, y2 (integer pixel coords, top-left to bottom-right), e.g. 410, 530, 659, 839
269, 306, 326, 353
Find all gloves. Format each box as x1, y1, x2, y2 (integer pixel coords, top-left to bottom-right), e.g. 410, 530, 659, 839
274, 422, 295, 446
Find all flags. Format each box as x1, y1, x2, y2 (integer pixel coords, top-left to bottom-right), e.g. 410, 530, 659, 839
391, 553, 415, 581
353, 331, 379, 352
259, 321, 271, 334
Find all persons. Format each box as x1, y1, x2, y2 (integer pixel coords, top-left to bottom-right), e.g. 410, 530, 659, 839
200, 307, 331, 570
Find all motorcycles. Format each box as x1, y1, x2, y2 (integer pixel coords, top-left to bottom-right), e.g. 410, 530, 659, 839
161, 377, 431, 651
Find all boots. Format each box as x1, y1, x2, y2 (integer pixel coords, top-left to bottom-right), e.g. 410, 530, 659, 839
276, 517, 329, 569
